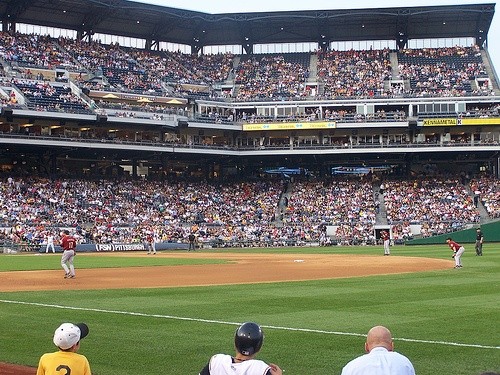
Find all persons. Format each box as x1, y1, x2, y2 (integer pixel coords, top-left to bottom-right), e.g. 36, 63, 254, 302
340, 326, 417, 375
446, 237, 465, 269
268, 363, 283, 375
199, 322, 272, 375
59, 230, 76, 278
0, 27, 500, 256
36, 323, 92, 375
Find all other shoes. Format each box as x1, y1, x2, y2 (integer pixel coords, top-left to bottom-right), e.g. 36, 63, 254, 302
475, 254, 482, 256
384, 254, 390, 256
153, 253, 156, 255
147, 252, 151, 254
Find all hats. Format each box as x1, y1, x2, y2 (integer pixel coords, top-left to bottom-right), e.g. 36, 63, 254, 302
52, 321, 90, 349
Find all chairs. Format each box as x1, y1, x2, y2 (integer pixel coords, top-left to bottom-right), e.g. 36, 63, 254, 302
0, 177, 500, 250
0, 29, 500, 118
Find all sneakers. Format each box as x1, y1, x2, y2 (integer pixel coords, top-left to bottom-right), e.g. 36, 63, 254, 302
454, 265, 463, 268
64, 272, 70, 278
68, 274, 75, 279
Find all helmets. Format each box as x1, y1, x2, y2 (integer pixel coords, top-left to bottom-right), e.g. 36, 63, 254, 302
234, 321, 264, 355
